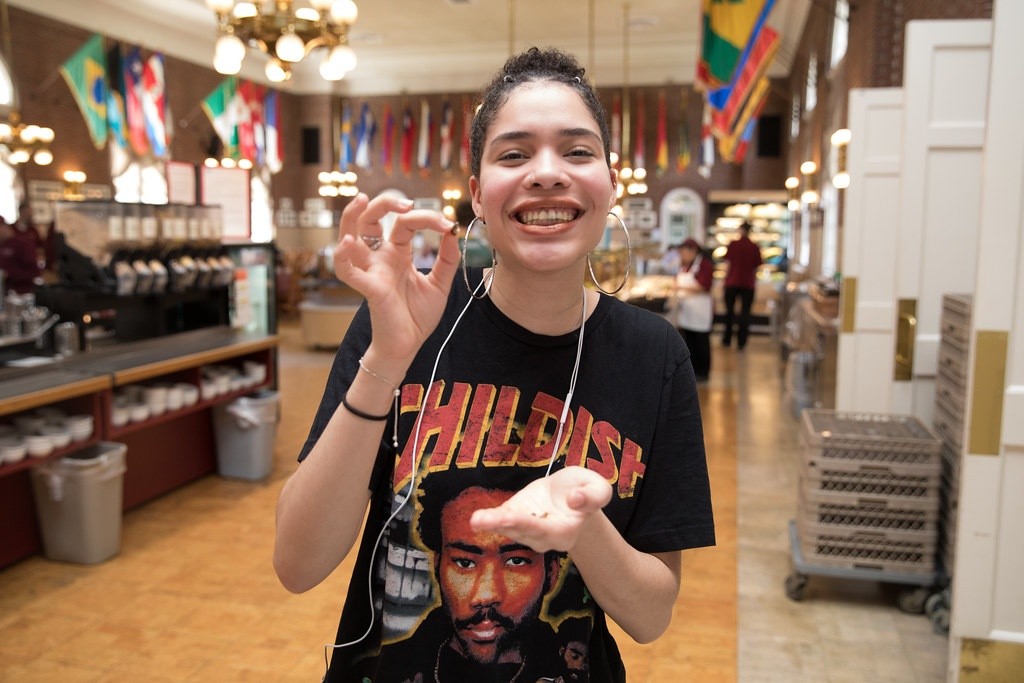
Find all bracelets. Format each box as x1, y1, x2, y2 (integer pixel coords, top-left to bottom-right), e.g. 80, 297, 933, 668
343, 392, 390, 420
359, 360, 406, 447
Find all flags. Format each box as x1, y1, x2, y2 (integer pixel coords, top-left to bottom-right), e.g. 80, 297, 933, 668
339, 97, 473, 173
609, 86, 691, 176
58, 34, 173, 158
201, 76, 284, 171
694, 0, 781, 163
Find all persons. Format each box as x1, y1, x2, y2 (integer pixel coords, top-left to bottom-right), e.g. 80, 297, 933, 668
455, 201, 494, 269
0, 205, 56, 286
722, 223, 761, 348
273, 46, 714, 683
673, 240, 714, 381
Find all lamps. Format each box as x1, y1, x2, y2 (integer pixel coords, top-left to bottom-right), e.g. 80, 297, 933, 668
319, 169, 361, 209
208, 0, 357, 83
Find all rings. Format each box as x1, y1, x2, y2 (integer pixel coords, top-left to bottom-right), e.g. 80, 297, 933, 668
363, 236, 384, 250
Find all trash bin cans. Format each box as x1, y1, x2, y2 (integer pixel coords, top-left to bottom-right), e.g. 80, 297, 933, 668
785, 349, 815, 420
214, 388, 280, 481
34, 440, 127, 563
